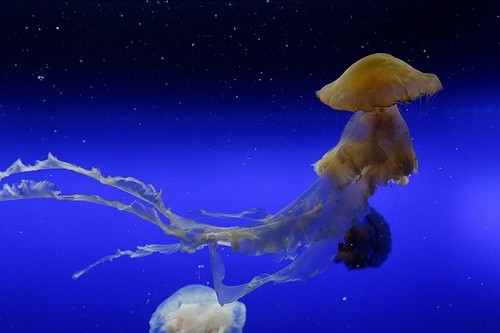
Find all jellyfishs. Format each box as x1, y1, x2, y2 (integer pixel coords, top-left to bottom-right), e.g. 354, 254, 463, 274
148, 284, 246, 333
0, 53, 443, 307
